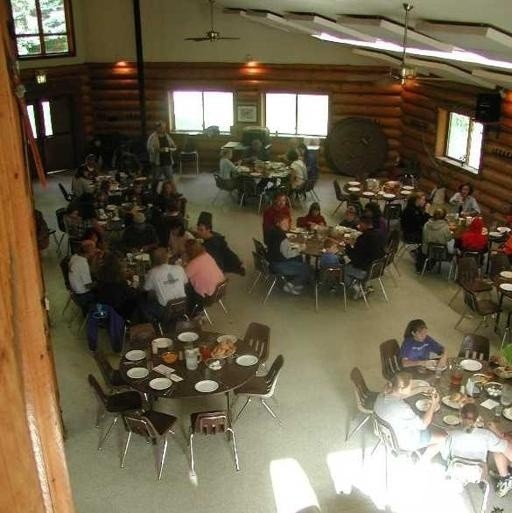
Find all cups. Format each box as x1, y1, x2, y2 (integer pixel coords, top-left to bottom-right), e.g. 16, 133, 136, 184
434, 356, 512, 416
459, 217, 472, 225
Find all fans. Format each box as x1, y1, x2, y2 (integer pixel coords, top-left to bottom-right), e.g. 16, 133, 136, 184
184, 0, 239, 42
383, 3, 447, 81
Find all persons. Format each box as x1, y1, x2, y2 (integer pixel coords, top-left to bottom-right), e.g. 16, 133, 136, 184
453, 217, 488, 266
217, 134, 319, 202
421, 209, 460, 274
448, 182, 482, 217
491, 343, 512, 369
263, 192, 391, 301
146, 118, 177, 199
399, 190, 435, 274
397, 318, 451, 369
490, 215, 512, 266
62, 154, 188, 257
492, 434, 512, 497
372, 372, 448, 465
440, 403, 510, 490
66, 211, 247, 354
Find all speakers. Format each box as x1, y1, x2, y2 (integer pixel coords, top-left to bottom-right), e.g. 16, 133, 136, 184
474, 92, 502, 123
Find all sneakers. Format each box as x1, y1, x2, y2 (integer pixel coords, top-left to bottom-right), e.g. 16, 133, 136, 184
495, 475, 512, 498
353, 288, 371, 301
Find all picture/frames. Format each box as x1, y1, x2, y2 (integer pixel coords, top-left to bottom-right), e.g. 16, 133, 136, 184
237, 104, 258, 123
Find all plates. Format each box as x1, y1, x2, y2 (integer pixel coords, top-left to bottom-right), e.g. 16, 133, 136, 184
497, 227, 511, 232
136, 254, 151, 261
499, 271, 512, 278
97, 203, 146, 228
125, 332, 258, 393
500, 283, 512, 291
348, 179, 415, 198
413, 359, 512, 425
489, 231, 504, 237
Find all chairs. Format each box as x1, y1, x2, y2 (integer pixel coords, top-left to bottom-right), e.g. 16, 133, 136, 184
88, 374, 142, 449
92, 348, 133, 392
350, 333, 512, 481
217, 153, 317, 213
120, 410, 177, 479
123, 323, 152, 353
55, 170, 229, 339
242, 322, 272, 358
249, 215, 398, 311
235, 355, 281, 421
332, 173, 511, 347
189, 411, 239, 478
